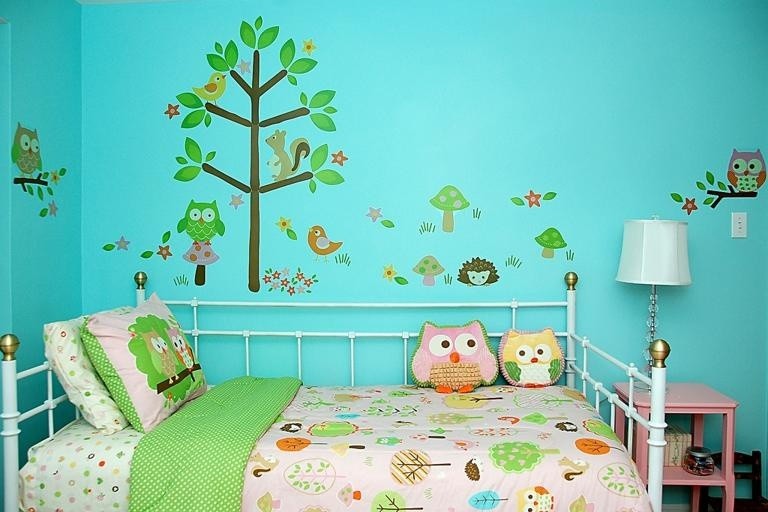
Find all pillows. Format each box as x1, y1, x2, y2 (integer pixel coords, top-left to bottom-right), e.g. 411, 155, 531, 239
79, 291, 208, 439
40, 304, 136, 437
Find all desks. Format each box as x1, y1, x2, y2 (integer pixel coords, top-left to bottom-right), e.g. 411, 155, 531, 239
609, 378, 739, 512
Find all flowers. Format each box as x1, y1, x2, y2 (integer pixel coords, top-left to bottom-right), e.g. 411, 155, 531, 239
261, 264, 321, 298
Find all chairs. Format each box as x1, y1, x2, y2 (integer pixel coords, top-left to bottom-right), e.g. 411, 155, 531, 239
702, 449, 766, 511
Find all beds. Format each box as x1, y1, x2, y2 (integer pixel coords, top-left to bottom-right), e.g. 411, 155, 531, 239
0, 270, 673, 510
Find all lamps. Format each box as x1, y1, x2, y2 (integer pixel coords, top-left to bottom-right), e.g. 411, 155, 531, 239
615, 214, 690, 389
